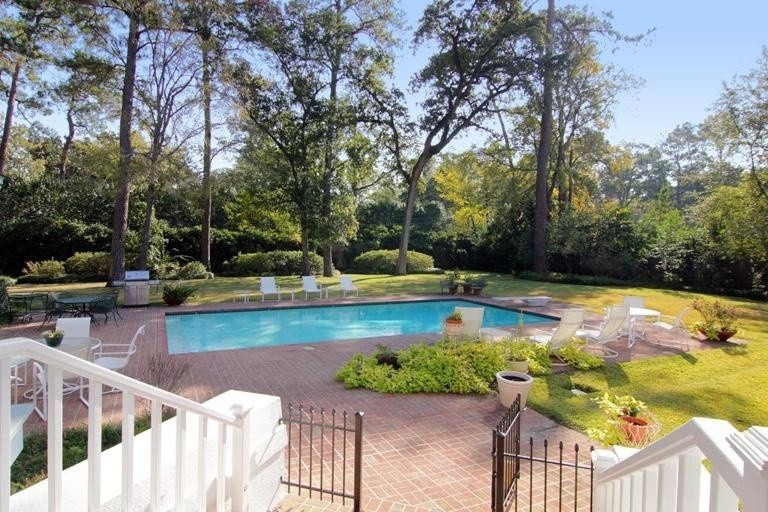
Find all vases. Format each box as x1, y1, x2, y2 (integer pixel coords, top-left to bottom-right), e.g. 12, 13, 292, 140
618, 416, 648, 442
496, 370, 533, 410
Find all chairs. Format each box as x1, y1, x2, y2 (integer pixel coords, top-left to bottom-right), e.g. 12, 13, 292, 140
260, 276, 281, 302
574, 304, 631, 358
303, 276, 321, 300
8, 290, 146, 422
619, 296, 646, 342
652, 307, 690, 353
529, 308, 585, 367
340, 276, 358, 298
455, 306, 485, 339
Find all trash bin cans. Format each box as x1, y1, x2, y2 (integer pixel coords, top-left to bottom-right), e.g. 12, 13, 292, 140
125, 286, 150, 305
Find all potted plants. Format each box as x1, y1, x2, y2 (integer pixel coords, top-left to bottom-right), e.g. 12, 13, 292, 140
163, 282, 197, 306
446, 269, 487, 295
444, 311, 463, 334
688, 298, 744, 342
507, 346, 529, 374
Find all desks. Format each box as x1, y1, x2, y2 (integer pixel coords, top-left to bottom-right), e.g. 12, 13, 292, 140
603, 306, 660, 341
113, 280, 159, 305
